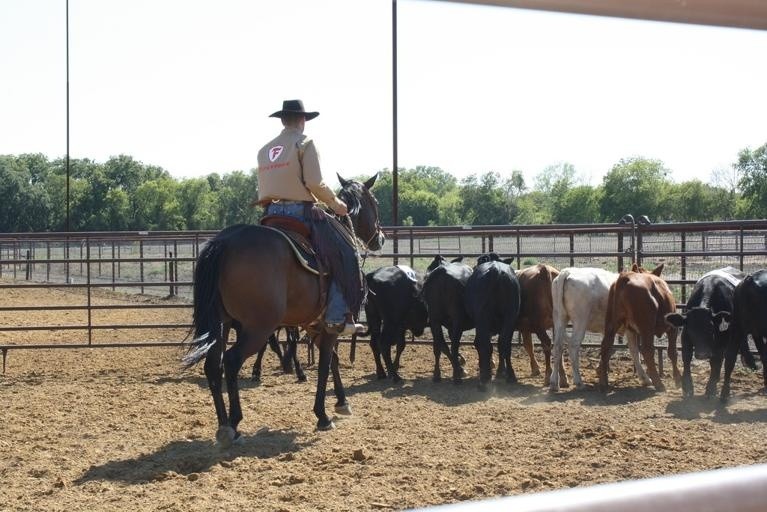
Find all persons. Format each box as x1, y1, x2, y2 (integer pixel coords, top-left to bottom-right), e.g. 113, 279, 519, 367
256, 100, 364, 335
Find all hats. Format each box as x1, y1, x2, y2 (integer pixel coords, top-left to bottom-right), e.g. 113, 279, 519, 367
266, 98, 320, 120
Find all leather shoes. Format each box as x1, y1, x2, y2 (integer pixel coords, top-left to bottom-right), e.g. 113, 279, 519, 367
323, 321, 363, 335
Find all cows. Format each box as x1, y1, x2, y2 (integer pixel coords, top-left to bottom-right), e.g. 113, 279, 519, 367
664, 266, 749, 401
414, 254, 476, 386
720, 269, 767, 404
549, 267, 653, 392
365, 265, 429, 386
598, 262, 681, 396
515, 262, 569, 388
251, 325, 307, 383
465, 252, 521, 393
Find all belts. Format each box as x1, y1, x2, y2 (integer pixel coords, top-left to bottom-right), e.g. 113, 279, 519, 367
270, 200, 303, 206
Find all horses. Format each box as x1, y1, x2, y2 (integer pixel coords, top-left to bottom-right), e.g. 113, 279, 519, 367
175, 173, 386, 445
